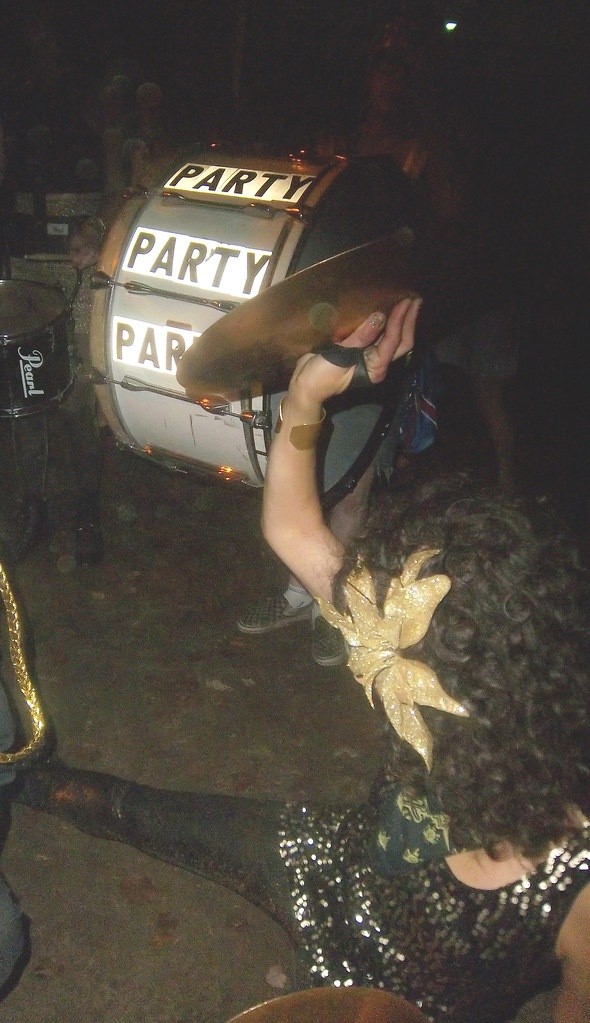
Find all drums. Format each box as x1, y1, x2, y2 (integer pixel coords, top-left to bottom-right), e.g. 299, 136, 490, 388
0, 277, 77, 419
87, 139, 401, 512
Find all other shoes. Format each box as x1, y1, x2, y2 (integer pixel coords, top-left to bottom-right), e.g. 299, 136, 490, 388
310, 606, 347, 666
236, 594, 314, 634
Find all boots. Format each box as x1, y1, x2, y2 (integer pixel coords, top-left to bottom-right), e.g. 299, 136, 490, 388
0, 496, 51, 566
4, 730, 137, 850
73, 498, 106, 565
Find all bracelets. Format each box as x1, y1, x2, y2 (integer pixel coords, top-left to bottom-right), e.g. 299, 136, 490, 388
84, 217, 107, 238
274, 394, 327, 450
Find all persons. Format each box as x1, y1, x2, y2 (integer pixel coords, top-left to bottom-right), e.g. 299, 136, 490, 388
1, 10, 131, 567
235, 400, 398, 667
7, 294, 590, 1023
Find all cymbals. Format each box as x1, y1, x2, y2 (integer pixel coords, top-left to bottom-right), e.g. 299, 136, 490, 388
176, 230, 419, 402
223, 984, 432, 1022
